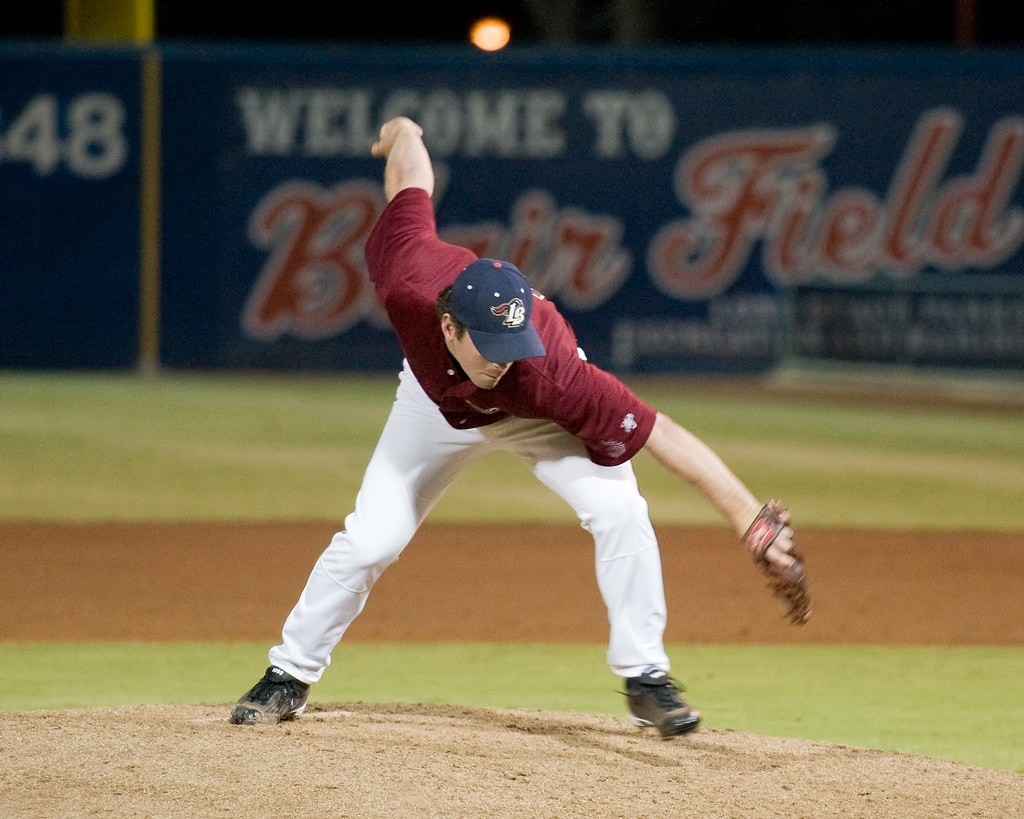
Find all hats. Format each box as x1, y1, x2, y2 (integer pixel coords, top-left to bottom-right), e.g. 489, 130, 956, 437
446, 258, 546, 364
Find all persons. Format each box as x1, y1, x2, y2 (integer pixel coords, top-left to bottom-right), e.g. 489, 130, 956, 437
228, 115, 813, 741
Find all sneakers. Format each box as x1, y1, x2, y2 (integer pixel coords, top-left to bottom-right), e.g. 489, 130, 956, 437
613, 666, 701, 739
229, 665, 310, 725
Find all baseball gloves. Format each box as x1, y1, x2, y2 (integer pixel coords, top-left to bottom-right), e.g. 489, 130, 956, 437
739, 498, 815, 628
